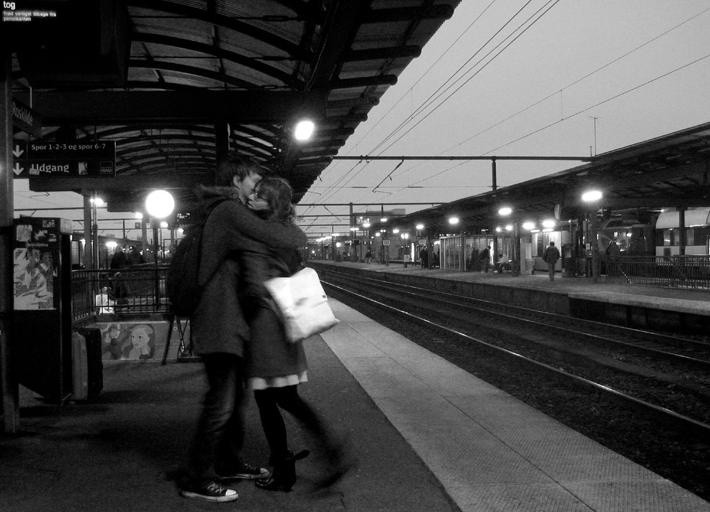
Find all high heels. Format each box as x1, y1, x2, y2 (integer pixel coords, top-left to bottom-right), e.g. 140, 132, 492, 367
255, 456, 296, 491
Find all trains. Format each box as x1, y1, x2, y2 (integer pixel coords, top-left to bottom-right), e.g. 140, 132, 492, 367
316, 207, 710, 280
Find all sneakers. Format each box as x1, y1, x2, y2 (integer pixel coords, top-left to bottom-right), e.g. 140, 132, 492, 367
215, 462, 271, 480
180, 482, 238, 502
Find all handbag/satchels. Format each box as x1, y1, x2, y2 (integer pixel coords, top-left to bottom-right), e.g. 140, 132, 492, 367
264, 264, 338, 344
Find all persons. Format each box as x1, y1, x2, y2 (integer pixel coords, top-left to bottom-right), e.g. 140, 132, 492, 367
238, 177, 351, 492
129, 324, 155, 360
365, 250, 371, 264
110, 244, 147, 268
466, 244, 491, 276
419, 245, 439, 270
542, 242, 560, 281
495, 254, 509, 274
574, 238, 622, 278
176, 154, 309, 505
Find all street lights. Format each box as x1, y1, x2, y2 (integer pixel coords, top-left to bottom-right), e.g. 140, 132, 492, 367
91, 197, 105, 313
145, 190, 177, 313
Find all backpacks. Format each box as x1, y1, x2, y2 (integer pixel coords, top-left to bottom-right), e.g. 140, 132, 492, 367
164, 197, 231, 316
